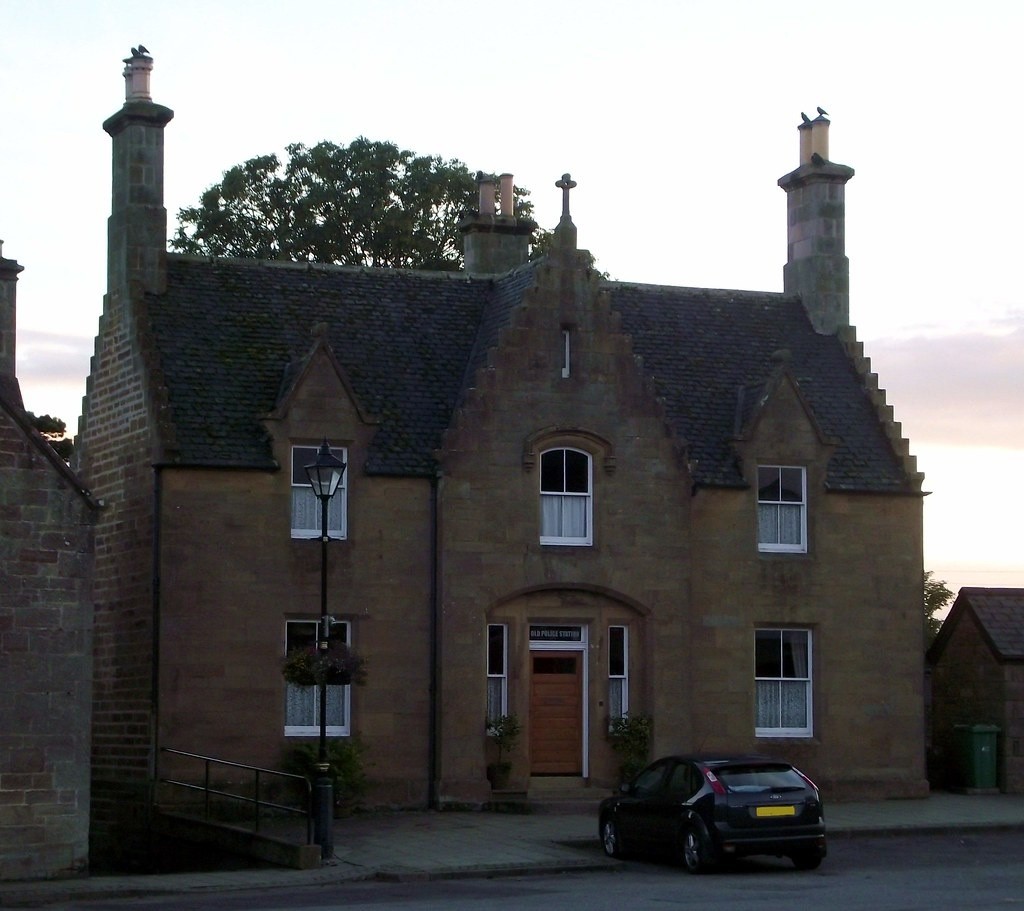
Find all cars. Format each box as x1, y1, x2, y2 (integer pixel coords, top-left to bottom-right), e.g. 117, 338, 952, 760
599, 752, 827, 869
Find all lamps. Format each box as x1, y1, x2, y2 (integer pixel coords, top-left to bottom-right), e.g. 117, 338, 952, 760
524, 438, 535, 473
603, 439, 616, 474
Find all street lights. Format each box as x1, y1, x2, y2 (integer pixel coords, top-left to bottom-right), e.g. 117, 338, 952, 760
302, 443, 344, 754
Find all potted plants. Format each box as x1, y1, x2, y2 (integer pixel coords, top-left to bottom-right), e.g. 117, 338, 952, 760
603, 712, 654, 775
487, 707, 524, 789
282, 732, 373, 819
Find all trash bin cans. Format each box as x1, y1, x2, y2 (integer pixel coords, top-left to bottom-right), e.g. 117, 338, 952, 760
952, 723, 1000, 788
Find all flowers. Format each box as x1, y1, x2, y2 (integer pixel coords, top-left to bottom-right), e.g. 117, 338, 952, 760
280, 634, 368, 686
